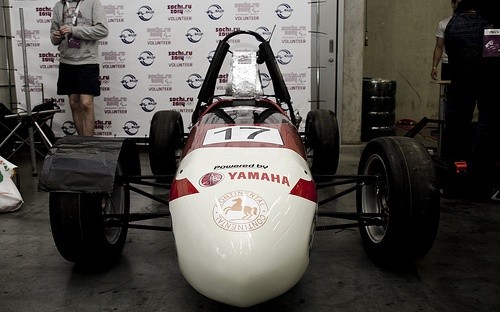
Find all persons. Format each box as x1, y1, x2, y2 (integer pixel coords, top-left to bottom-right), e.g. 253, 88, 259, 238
50, 0, 109, 137
439, 0, 500, 203
431, 0, 460, 80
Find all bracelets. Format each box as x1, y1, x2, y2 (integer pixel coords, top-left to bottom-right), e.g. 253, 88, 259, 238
433, 67, 437, 69
